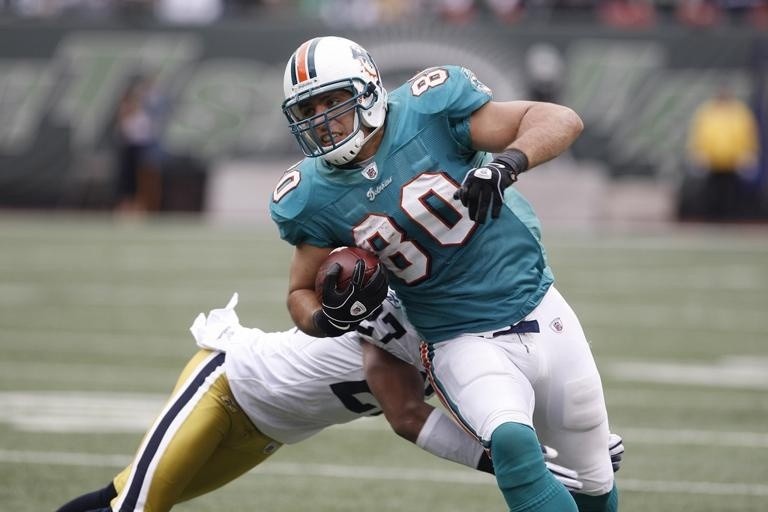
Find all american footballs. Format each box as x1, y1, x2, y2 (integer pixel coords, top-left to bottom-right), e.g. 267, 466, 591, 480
315, 246, 381, 307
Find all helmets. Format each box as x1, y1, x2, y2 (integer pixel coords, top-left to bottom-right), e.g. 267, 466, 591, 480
278, 36, 387, 170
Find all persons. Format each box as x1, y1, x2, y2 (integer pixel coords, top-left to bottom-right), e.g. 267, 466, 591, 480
49, 281, 627, 512
270, 34, 623, 512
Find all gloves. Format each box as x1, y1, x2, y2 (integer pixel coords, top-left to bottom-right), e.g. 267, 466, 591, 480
317, 258, 391, 336
452, 159, 518, 226
475, 431, 625, 497
